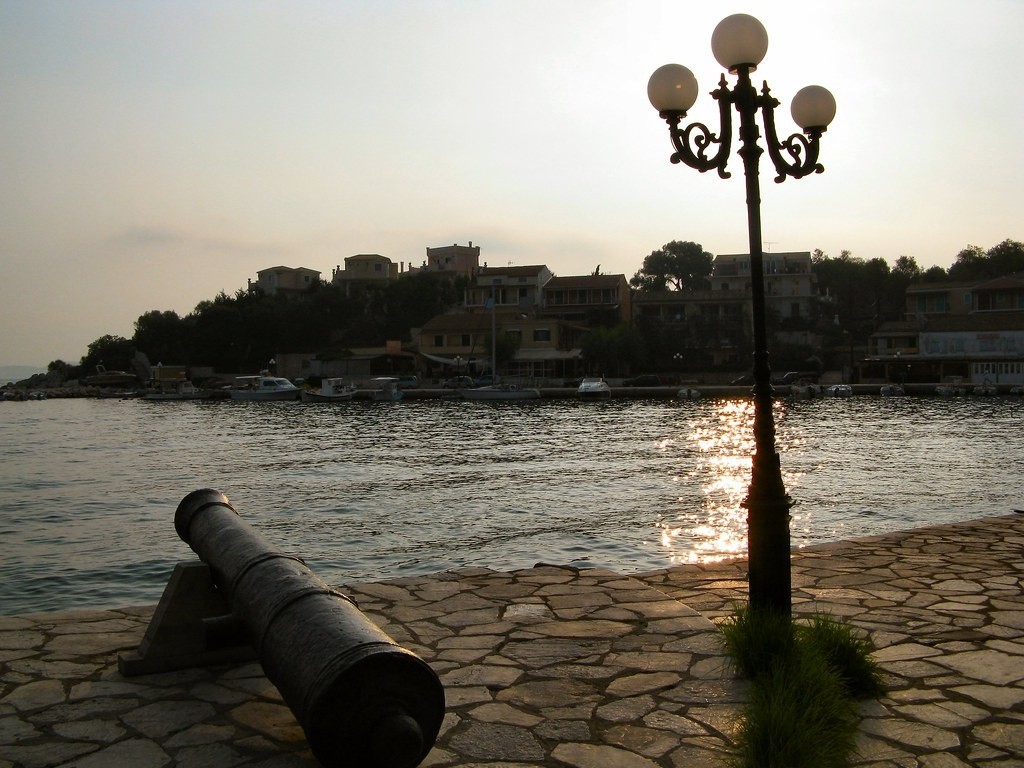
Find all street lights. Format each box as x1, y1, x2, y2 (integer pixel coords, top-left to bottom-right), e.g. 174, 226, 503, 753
673, 352, 684, 386
893, 351, 901, 383
646, 13, 840, 635
454, 355, 463, 388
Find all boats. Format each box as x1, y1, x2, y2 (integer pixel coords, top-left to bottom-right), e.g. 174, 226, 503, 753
576, 376, 612, 401
933, 384, 967, 398
823, 384, 853, 397
140, 363, 213, 401
1009, 384, 1024, 396
973, 377, 1000, 397
879, 383, 905, 397
676, 388, 701, 400
301, 377, 360, 403
368, 376, 404, 402
222, 374, 302, 402
88, 364, 140, 399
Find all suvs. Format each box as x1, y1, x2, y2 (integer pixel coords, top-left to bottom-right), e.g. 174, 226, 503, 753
623, 374, 700, 387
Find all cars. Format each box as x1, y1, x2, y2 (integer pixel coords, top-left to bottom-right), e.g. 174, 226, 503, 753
442, 374, 503, 389
398, 375, 420, 389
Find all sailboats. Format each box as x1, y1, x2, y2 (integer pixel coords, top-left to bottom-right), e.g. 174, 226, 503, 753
454, 289, 545, 402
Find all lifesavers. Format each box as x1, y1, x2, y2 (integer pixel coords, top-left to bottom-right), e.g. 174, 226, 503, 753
251, 387, 256, 392
600, 384, 604, 389
509, 385, 516, 391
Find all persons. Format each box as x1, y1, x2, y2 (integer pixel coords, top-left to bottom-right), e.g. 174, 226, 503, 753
496, 373, 513, 385
528, 372, 534, 387
269, 365, 277, 377
478, 368, 485, 377
983, 368, 989, 384
438, 368, 446, 389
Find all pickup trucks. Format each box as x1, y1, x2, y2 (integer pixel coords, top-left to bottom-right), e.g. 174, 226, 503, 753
773, 371, 817, 386
